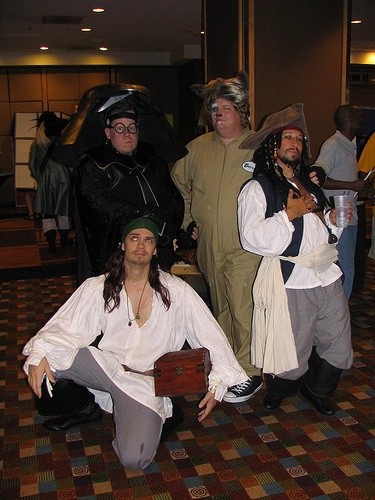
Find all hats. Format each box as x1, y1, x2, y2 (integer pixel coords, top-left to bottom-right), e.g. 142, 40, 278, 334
239, 103, 315, 160
38, 111, 68, 136
122, 217, 159, 244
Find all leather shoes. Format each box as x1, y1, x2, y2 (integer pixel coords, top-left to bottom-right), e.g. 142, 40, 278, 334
262, 393, 283, 411
167, 400, 185, 424
44, 404, 101, 432
301, 381, 337, 417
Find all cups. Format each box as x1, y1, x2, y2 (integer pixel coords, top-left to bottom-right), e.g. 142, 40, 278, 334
334, 195, 350, 228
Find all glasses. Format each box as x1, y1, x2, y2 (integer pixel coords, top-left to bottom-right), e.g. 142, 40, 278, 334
110, 123, 140, 134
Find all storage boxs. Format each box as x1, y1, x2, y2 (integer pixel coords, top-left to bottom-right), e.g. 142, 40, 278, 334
154, 348, 212, 397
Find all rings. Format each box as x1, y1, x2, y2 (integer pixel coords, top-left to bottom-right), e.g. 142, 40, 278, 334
301, 195, 307, 200
306, 204, 312, 208
304, 200, 310, 204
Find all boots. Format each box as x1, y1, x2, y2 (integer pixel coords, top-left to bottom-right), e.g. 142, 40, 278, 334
44, 229, 56, 250
60, 229, 73, 246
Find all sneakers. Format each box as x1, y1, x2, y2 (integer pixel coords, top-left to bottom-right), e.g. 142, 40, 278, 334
222, 377, 263, 403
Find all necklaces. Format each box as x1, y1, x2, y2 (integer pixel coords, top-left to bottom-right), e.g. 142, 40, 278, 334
123, 279, 148, 327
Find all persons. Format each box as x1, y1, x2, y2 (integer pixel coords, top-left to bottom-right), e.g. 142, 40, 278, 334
67, 103, 185, 286
237, 103, 354, 416
21, 217, 249, 471
29, 111, 75, 251
310, 104, 375, 302
171, 72, 263, 403
